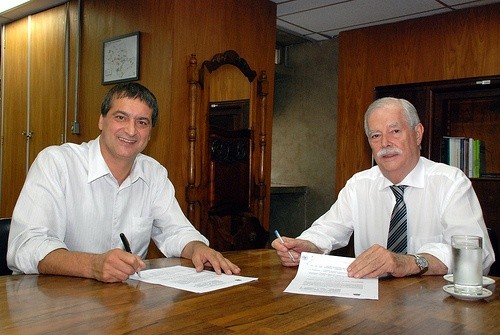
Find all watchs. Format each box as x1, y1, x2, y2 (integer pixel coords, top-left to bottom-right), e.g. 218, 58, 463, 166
406, 253, 429, 276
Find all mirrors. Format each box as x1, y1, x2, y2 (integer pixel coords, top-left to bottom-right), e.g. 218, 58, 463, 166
188, 48, 269, 251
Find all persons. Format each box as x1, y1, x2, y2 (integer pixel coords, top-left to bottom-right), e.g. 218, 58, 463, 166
272, 96, 495, 279
8, 81, 241, 283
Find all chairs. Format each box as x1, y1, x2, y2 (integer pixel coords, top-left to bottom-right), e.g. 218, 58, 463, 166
0, 217, 13, 275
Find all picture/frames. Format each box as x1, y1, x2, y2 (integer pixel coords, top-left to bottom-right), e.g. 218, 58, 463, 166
101, 30, 139, 84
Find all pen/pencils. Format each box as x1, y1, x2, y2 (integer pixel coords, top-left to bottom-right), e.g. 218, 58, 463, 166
119, 232, 142, 280
274, 230, 296, 263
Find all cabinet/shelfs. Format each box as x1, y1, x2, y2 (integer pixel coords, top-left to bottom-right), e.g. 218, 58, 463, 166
208, 99, 251, 215
375, 74, 500, 181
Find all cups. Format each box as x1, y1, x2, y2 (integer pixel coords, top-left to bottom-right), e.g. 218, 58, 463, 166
450, 235, 484, 296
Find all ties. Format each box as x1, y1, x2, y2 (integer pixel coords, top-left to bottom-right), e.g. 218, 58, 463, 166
387, 185, 407, 258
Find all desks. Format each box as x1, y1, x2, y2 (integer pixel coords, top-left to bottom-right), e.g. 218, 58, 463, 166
0, 248, 500, 335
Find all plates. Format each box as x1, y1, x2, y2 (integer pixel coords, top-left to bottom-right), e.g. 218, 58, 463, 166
444, 274, 496, 287
443, 284, 493, 301
